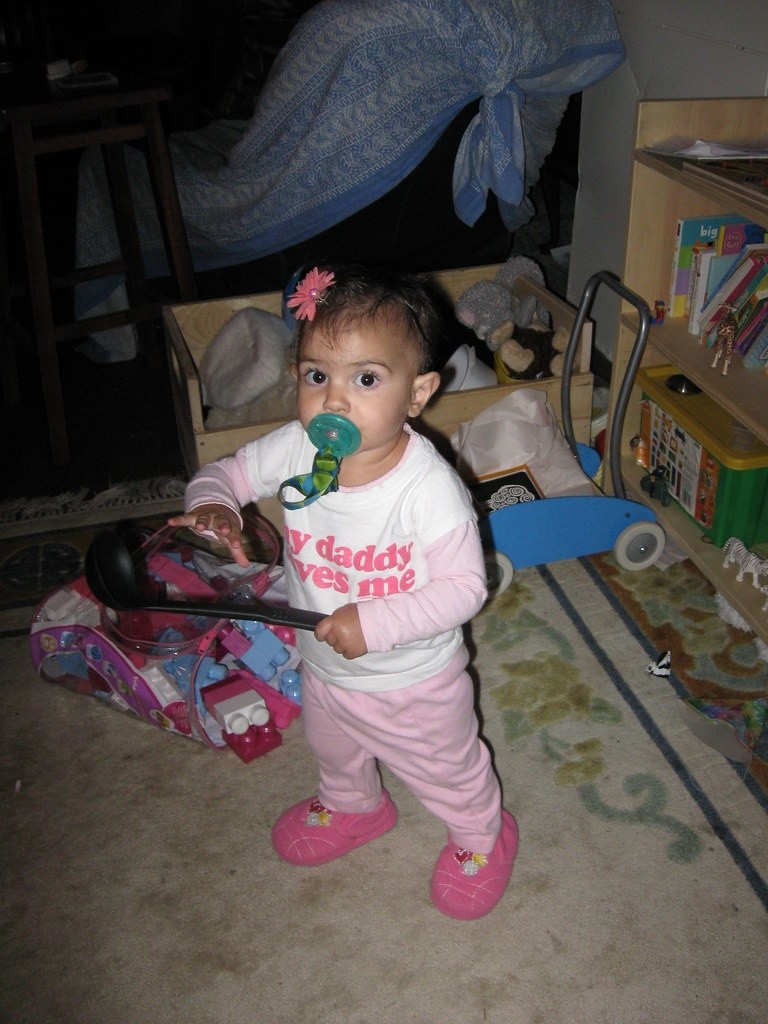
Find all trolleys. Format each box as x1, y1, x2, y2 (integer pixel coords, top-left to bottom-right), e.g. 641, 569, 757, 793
465, 273, 665, 601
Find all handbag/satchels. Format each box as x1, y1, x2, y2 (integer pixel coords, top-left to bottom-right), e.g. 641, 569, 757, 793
30, 512, 304, 752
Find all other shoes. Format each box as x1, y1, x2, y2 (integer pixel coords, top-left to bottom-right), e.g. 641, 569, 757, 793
431, 808, 518, 919
272, 788, 398, 867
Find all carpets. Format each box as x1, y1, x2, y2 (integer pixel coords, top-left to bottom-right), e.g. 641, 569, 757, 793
0, 459, 767, 1024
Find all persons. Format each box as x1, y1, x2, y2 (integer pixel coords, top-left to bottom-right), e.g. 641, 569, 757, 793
168, 267, 518, 922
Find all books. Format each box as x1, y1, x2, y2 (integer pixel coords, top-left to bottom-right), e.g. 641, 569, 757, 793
670, 213, 768, 368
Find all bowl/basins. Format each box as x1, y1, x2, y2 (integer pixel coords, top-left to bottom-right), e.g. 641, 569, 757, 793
437, 343, 498, 393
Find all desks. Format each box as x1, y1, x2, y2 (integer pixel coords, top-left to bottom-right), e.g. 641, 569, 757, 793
1, 68, 205, 480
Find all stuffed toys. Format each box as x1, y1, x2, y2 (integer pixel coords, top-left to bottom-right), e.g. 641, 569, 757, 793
197, 256, 578, 437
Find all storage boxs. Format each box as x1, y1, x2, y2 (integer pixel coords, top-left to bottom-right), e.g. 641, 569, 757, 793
164, 261, 594, 490
634, 366, 767, 550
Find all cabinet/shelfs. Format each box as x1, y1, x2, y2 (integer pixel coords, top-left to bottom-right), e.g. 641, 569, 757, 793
600, 96, 768, 651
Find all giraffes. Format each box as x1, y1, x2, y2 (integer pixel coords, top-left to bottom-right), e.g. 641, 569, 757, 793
708, 302, 740, 378
